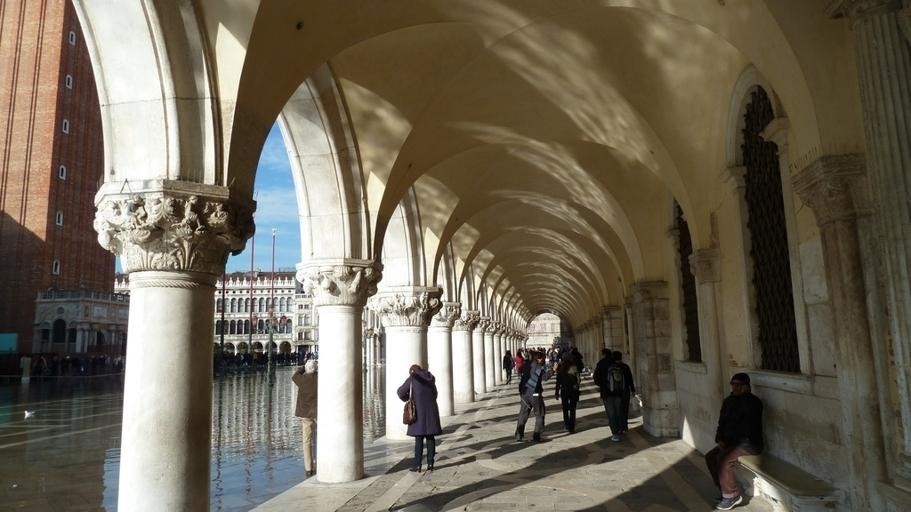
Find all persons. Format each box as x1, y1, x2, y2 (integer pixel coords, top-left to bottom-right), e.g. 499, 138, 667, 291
291, 358, 317, 478
556, 351, 580, 434
396, 364, 443, 472
502, 346, 585, 386
594, 347, 626, 443
705, 373, 765, 509
612, 350, 637, 433
515, 351, 560, 443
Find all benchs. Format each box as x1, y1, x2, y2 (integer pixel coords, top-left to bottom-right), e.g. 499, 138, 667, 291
738, 453, 837, 512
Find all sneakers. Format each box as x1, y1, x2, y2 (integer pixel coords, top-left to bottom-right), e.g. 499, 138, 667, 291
712, 494, 744, 510
514, 424, 629, 442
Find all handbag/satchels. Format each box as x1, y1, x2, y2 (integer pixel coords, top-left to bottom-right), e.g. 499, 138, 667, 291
401, 400, 418, 424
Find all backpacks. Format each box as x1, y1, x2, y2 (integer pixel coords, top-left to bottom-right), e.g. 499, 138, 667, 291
607, 360, 630, 394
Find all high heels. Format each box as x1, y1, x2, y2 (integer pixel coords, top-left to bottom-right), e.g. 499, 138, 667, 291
410, 465, 422, 473
428, 462, 435, 470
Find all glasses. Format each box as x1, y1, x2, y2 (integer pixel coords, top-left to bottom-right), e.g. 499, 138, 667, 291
730, 381, 747, 387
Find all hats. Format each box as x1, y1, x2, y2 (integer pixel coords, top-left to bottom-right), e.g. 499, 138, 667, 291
732, 372, 751, 385
